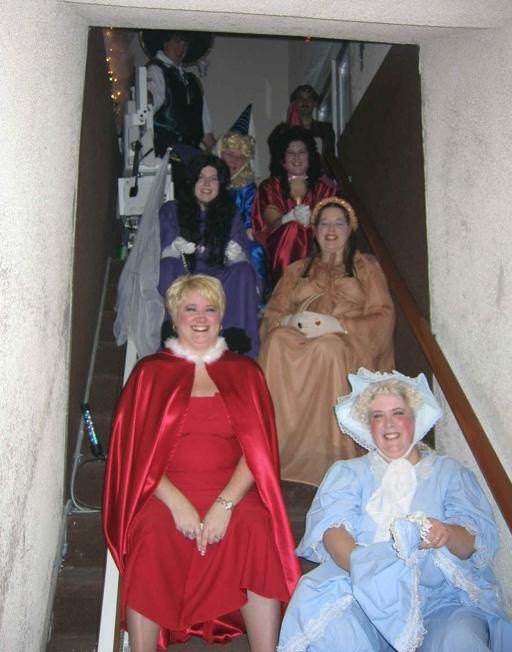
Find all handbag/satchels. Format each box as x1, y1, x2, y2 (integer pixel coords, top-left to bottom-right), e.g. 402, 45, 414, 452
116, 172, 171, 219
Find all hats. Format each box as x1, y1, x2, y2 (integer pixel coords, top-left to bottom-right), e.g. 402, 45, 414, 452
138, 29, 215, 66
290, 86, 318, 101
228, 102, 256, 136
331, 363, 442, 462
